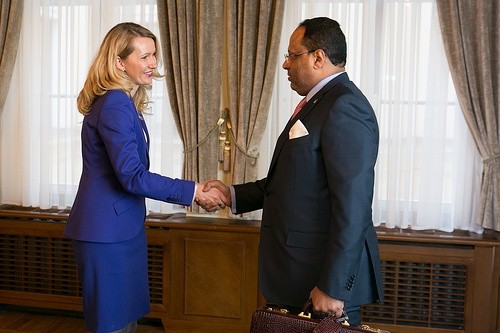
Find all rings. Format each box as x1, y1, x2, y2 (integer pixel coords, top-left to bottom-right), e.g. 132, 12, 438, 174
217, 202, 222, 208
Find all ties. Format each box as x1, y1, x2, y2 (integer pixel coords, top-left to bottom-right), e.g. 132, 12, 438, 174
289, 96, 307, 119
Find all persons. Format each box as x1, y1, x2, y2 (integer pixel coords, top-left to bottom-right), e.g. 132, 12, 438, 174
194, 16, 384, 333
64, 22, 231, 333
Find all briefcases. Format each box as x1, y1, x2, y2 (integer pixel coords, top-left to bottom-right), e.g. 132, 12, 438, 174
250, 298, 390, 333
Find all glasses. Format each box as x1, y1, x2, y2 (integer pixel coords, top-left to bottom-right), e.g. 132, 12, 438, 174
283, 49, 315, 60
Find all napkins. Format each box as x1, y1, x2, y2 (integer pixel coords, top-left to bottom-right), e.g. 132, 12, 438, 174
287, 119, 309, 140
